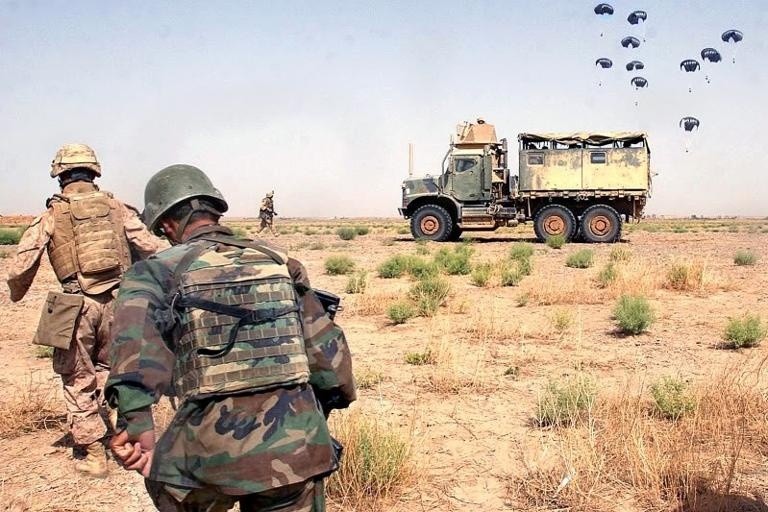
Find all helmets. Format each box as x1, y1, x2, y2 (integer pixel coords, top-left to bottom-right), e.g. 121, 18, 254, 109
144, 164, 228, 237
266, 192, 273, 198
50, 143, 101, 178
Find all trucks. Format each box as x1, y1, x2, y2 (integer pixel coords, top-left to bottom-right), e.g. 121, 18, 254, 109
398, 120, 654, 244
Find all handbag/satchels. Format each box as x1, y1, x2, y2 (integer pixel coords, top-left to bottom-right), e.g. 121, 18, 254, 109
32, 290, 84, 350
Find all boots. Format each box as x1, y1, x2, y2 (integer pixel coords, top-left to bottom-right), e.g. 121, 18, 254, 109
74, 441, 107, 479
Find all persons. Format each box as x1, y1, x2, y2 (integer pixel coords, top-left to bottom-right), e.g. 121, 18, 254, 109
6, 143, 159, 478
255, 192, 280, 237
105, 164, 357, 512
